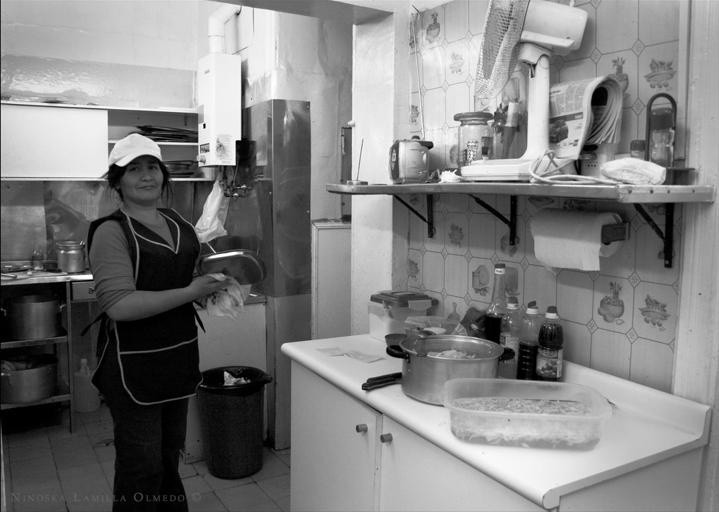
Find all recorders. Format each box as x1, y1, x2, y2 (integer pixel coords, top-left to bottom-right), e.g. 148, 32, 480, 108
389, 142, 430, 183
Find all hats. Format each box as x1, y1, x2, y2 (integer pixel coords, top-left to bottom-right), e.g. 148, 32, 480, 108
109, 132, 164, 168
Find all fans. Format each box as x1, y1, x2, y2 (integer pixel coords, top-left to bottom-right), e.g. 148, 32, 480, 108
461, 0, 590, 183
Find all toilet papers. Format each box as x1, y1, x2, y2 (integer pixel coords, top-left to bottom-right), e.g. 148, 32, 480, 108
528, 208, 626, 271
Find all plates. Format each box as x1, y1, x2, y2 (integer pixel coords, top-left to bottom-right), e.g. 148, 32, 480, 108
197, 249, 268, 286
137, 124, 198, 144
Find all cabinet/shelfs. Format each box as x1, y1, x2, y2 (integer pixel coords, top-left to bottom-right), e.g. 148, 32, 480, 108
281, 322, 712, 511
2, 277, 86, 435
0, 100, 200, 185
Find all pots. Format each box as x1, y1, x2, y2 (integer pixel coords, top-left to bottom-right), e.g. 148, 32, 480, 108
386, 335, 515, 407
0, 351, 57, 404
0, 295, 67, 339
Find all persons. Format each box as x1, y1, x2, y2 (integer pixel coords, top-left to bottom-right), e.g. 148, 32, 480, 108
88, 133, 232, 512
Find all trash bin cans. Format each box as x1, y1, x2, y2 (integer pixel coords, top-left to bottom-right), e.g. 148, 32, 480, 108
194, 365, 274, 480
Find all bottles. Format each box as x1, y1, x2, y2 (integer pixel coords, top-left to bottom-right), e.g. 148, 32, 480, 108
534, 306, 563, 381
485, 264, 506, 344
516, 301, 542, 381
499, 296, 521, 379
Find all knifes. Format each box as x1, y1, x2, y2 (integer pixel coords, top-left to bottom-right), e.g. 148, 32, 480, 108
362, 372, 403, 391
